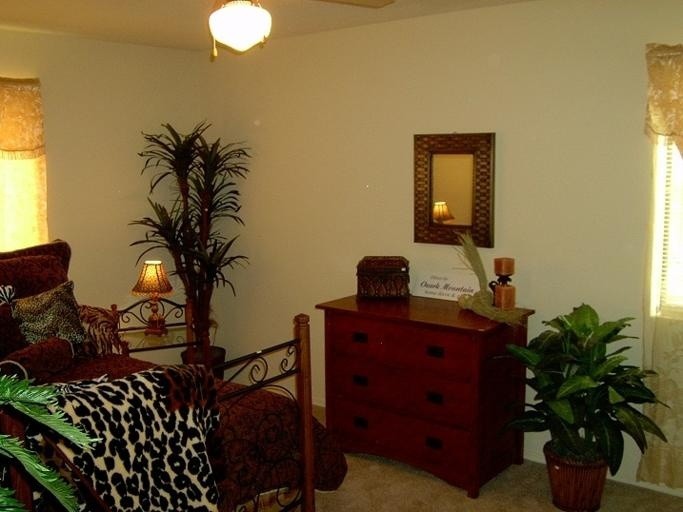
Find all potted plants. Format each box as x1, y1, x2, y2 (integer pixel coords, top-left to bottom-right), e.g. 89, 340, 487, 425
127, 120, 253, 395
506, 303, 671, 512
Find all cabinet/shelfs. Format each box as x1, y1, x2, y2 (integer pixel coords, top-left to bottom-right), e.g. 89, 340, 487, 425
315, 293, 534, 500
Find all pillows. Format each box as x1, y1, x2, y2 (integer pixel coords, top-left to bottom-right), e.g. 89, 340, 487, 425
0, 239, 72, 298
78, 304, 128, 358
13, 281, 89, 346
0, 337, 75, 384
19, 296, 86, 348
0, 302, 26, 361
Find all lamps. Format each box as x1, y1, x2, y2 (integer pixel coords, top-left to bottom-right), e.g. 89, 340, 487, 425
205, 0, 273, 61
432, 202, 454, 222
132, 261, 171, 337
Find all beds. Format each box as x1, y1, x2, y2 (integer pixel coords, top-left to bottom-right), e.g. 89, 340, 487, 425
0, 239, 348, 512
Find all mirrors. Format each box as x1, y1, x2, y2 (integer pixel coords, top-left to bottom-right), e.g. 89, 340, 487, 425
413, 132, 495, 248
